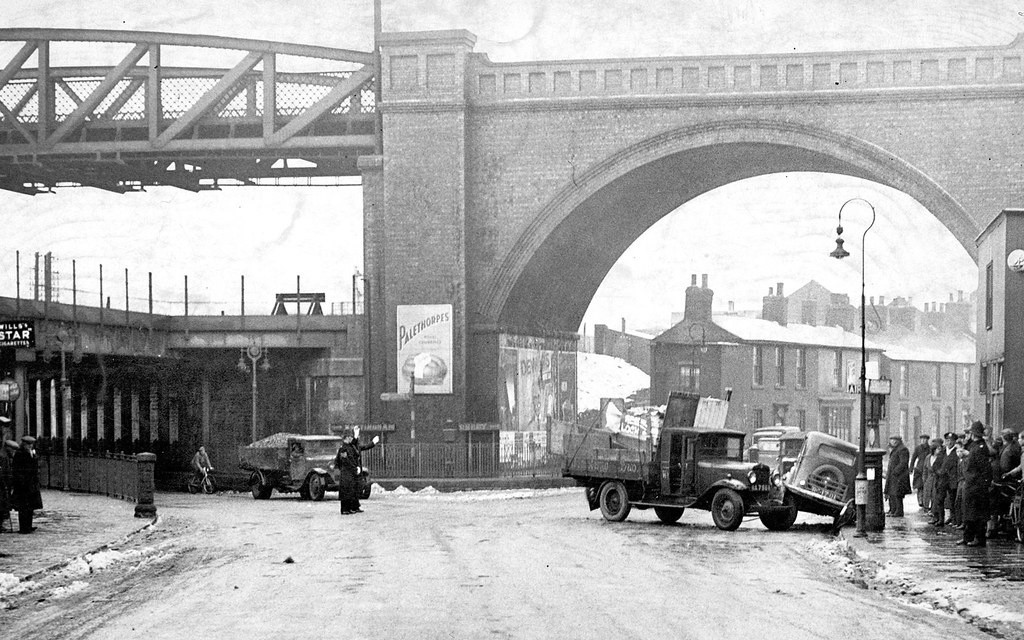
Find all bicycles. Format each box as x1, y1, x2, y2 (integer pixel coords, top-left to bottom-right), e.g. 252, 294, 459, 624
187, 468, 218, 494
1001, 474, 1024, 542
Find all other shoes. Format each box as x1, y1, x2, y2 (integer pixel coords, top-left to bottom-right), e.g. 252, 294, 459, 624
0, 525, 13, 533
956, 537, 974, 545
967, 539, 985, 547
342, 508, 364, 514
919, 500, 965, 529
885, 510, 904, 517
20, 527, 37, 533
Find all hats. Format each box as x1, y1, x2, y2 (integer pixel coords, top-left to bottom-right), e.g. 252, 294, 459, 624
958, 434, 965, 438
1001, 428, 1015, 440
920, 435, 930, 439
21, 436, 36, 442
199, 446, 205, 451
890, 435, 902, 439
944, 432, 958, 440
5, 440, 20, 449
969, 421, 985, 436
964, 429, 970, 433
932, 438, 944, 443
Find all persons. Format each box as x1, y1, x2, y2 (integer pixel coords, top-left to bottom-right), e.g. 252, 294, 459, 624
884, 435, 912, 517
12, 436, 43, 534
909, 421, 1024, 547
190, 446, 211, 486
333, 425, 379, 515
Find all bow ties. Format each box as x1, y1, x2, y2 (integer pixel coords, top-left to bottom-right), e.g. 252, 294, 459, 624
29, 449, 36, 458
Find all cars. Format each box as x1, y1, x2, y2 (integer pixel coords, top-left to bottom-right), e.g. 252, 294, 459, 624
778, 429, 862, 536
743, 425, 808, 474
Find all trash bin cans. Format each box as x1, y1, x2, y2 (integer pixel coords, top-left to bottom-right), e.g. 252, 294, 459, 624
134, 452, 157, 518
859, 447, 887, 533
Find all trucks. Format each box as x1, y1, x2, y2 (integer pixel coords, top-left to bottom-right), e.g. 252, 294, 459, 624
239, 434, 371, 503
562, 427, 798, 532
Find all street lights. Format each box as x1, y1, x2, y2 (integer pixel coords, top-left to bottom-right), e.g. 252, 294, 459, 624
56, 320, 71, 493
829, 196, 876, 537
244, 339, 263, 442
